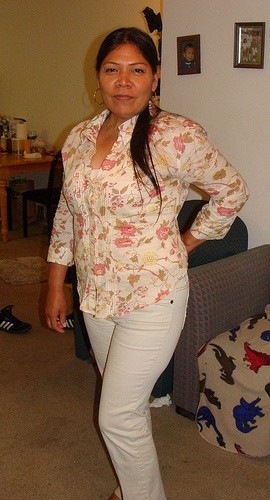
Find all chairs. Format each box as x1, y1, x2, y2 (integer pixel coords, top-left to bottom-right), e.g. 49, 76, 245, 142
23, 149, 64, 238
73, 200, 248, 398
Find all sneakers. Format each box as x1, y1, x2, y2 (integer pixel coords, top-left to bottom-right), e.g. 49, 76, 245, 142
64, 314, 74, 329
0, 305, 33, 335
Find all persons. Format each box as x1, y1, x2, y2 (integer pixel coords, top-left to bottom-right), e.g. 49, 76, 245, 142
181, 43, 195, 69
44, 28, 249, 500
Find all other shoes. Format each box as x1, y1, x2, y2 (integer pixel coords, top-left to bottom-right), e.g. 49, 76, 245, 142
110, 485, 122, 500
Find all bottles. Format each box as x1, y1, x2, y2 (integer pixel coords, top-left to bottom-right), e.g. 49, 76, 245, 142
0, 132, 6, 151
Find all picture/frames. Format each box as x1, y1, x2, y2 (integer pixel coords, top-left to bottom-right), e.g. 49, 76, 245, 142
233, 21, 265, 69
176, 34, 200, 75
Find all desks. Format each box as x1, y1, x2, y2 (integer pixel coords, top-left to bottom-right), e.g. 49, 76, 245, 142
0, 153, 65, 242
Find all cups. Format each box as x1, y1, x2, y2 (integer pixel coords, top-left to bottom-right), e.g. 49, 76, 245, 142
11, 138, 24, 156
27, 129, 38, 142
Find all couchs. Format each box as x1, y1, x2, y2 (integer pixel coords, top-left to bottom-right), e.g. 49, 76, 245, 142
173, 242, 270, 457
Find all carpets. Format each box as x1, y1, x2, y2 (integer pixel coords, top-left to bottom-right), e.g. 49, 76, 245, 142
0, 256, 49, 285
